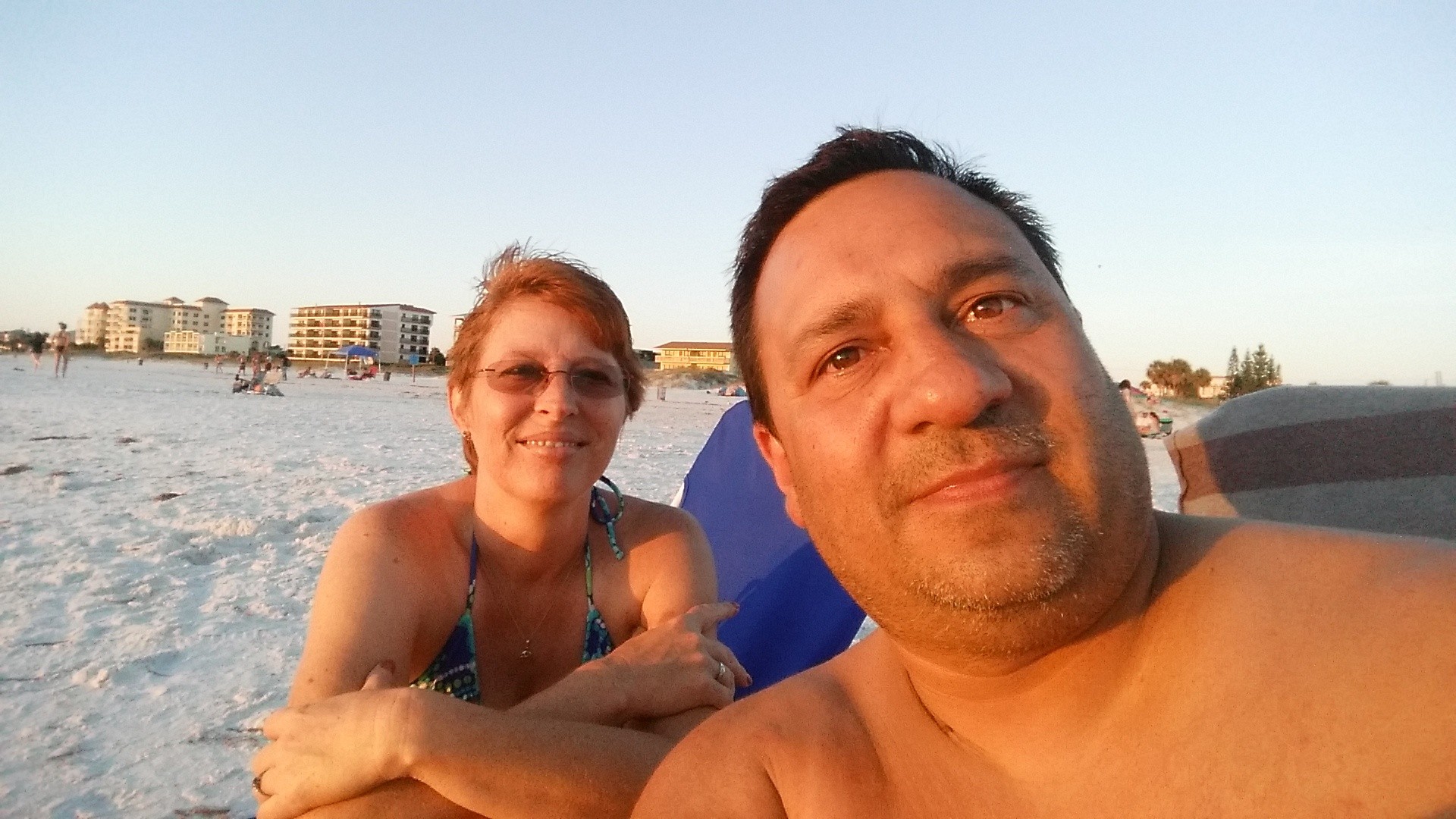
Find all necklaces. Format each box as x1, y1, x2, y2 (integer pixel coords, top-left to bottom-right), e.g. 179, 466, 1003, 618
479, 549, 581, 660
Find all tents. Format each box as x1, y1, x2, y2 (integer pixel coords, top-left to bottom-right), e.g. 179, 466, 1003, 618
324, 344, 381, 381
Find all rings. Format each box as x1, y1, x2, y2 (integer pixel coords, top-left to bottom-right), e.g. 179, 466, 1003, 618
716, 662, 725, 681
252, 770, 272, 797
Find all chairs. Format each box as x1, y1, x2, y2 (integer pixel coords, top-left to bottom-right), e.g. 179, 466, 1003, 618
664, 385, 1456, 702
349, 365, 378, 381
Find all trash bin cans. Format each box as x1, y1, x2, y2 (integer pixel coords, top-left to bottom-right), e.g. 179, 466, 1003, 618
1159, 418, 1173, 434
138, 359, 143, 366
384, 372, 391, 381
656, 387, 667, 400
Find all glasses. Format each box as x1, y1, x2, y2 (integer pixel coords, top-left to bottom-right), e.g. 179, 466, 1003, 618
464, 359, 634, 400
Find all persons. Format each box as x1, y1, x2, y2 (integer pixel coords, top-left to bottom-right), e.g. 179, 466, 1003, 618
1119, 379, 1159, 435
348, 370, 353, 374
51, 323, 69, 377
235, 351, 288, 380
363, 368, 367, 373
215, 353, 223, 374
630, 127, 1456, 819
31, 333, 43, 369
252, 238, 753, 819
